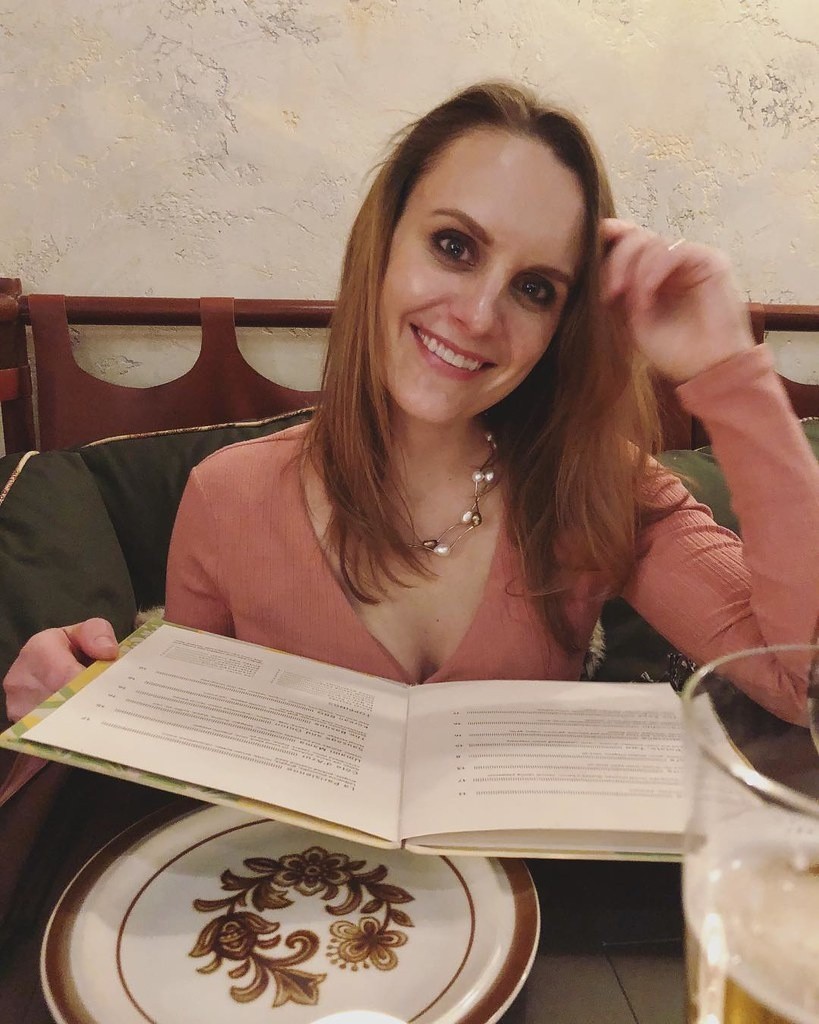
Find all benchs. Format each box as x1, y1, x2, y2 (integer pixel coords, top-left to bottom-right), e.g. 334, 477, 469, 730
0, 276, 818, 780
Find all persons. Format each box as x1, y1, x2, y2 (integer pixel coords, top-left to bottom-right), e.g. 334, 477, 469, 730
2, 84, 819, 727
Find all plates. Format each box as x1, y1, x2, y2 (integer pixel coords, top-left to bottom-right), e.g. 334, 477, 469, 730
40, 798, 541, 1024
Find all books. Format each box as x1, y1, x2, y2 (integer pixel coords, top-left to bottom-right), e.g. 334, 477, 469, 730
0, 617, 757, 865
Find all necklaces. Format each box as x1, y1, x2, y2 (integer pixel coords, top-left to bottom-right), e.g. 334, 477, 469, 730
404, 431, 498, 559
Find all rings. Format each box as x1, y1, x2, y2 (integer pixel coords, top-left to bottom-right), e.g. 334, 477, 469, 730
663, 235, 688, 269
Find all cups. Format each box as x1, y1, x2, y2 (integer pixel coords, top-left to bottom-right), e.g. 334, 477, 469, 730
681, 645, 819, 1024
